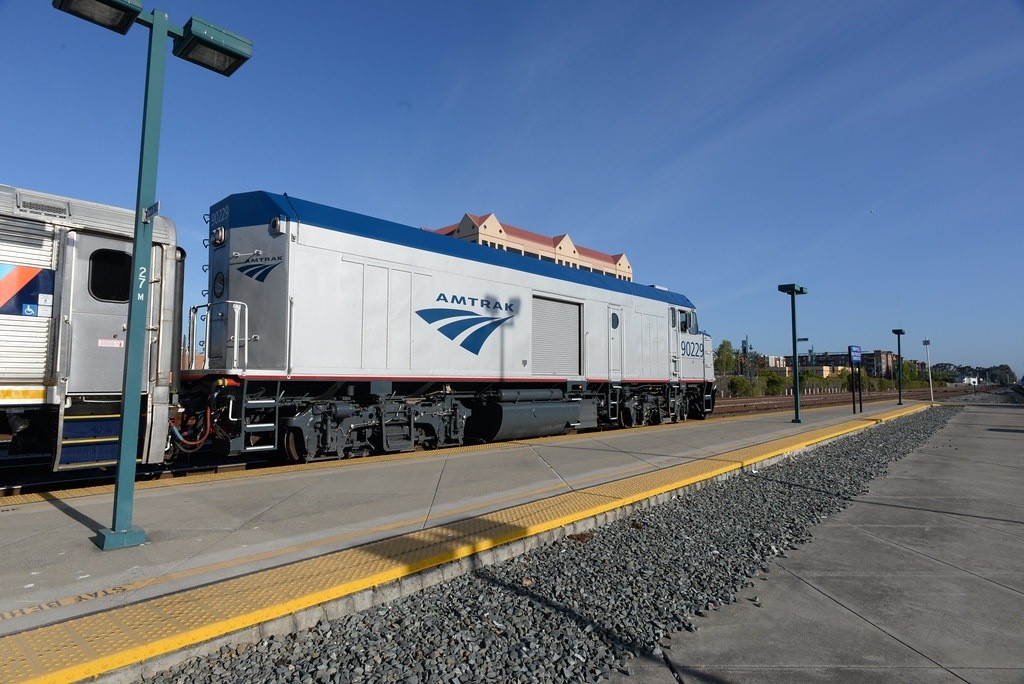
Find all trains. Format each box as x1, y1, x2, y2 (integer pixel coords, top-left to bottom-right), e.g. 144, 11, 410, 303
0, 183, 719, 475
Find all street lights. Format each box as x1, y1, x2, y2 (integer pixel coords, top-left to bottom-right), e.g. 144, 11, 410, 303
892, 329, 906, 405
778, 284, 808, 423
51, 1, 254, 551
